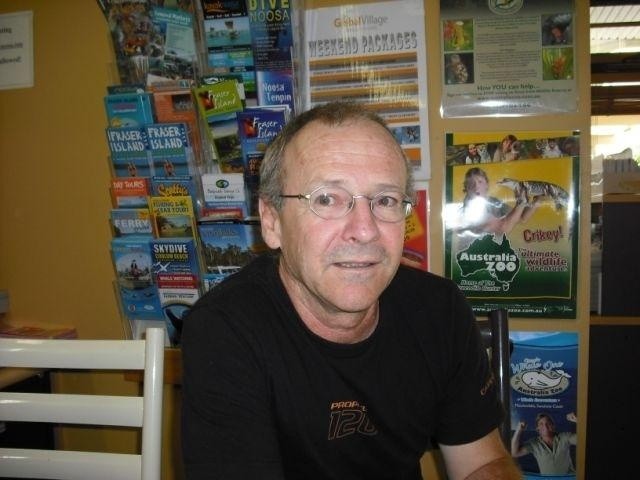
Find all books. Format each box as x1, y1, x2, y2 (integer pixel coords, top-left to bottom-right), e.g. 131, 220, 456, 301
98, 2, 297, 348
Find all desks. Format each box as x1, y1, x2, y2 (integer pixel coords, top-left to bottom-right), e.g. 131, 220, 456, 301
0, 323, 77, 449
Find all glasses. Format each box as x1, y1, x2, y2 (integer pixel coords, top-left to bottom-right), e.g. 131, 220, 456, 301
277, 185, 415, 224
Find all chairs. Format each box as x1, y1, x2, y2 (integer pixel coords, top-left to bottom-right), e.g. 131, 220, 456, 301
476, 309, 511, 455
0, 327, 165, 480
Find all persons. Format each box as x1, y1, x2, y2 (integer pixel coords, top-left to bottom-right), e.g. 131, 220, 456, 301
179, 102, 523, 479
509, 411, 577, 477
492, 135, 518, 163
542, 138, 562, 158
465, 144, 480, 164
461, 166, 544, 242
